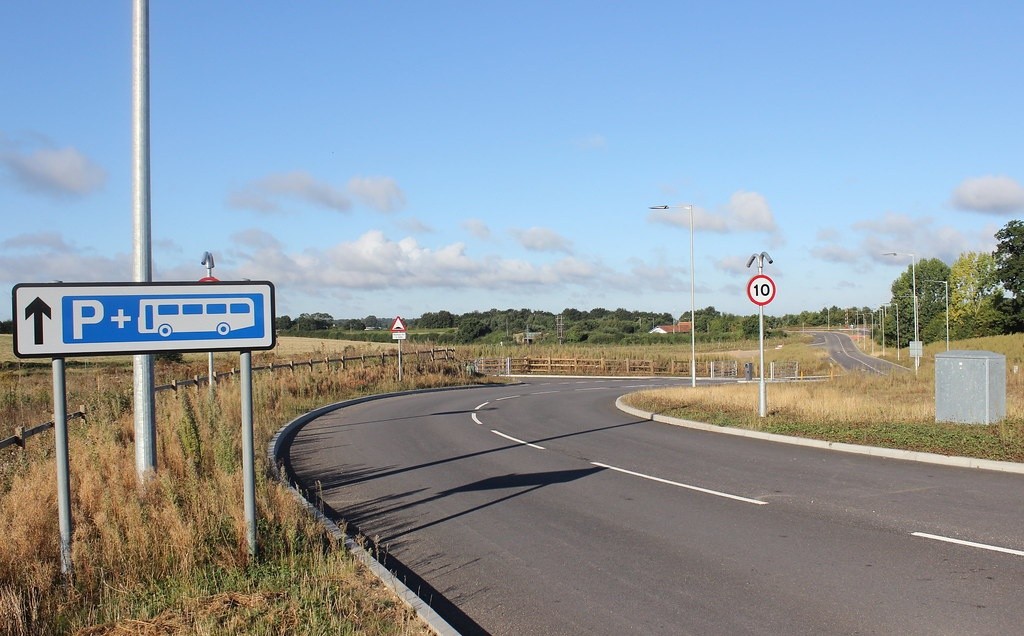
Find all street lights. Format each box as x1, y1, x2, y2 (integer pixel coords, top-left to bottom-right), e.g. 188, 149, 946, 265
847, 254, 951, 374
649, 204, 696, 387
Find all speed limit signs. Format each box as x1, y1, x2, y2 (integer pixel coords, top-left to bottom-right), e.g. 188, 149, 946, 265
747, 275, 776, 306
